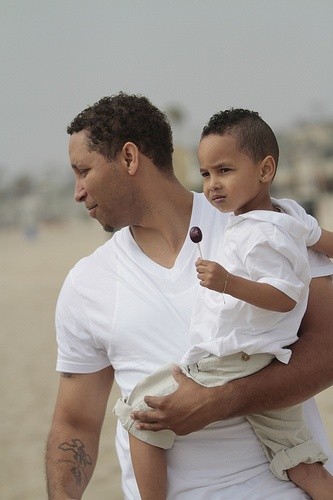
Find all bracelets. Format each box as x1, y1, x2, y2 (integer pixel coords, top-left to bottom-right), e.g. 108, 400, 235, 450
221, 271, 232, 295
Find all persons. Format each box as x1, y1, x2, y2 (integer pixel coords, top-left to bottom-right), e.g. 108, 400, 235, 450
44, 91, 332, 500
106, 108, 333, 500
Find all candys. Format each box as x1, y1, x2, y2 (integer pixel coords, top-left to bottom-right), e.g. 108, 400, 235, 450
190, 226, 202, 243
